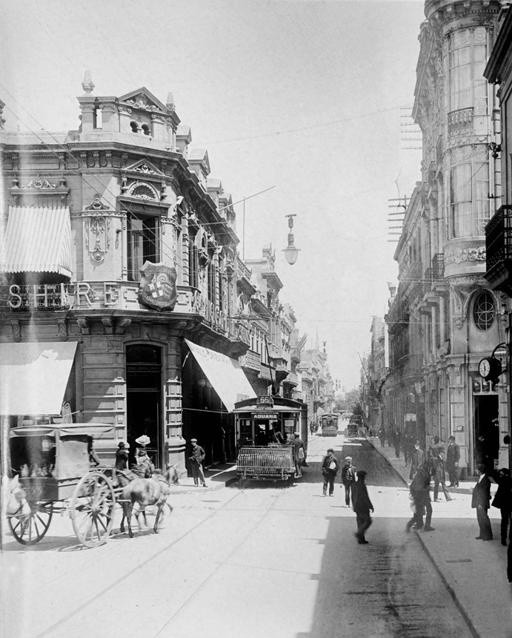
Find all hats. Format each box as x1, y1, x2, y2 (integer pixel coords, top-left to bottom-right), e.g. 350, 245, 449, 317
135, 435, 151, 446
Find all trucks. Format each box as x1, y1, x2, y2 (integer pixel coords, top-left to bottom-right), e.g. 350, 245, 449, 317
230, 393, 311, 484
317, 410, 342, 437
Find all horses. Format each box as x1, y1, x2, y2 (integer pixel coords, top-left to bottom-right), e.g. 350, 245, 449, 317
106, 456, 153, 535
119, 462, 181, 538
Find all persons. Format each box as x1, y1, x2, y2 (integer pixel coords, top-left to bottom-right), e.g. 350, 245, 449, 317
188, 438, 208, 487
321, 449, 339, 497
352, 471, 374, 544
271, 421, 306, 478
491, 468, 511, 547
88, 436, 100, 466
472, 463, 493, 541
341, 456, 357, 508
114, 442, 155, 480
377, 424, 460, 533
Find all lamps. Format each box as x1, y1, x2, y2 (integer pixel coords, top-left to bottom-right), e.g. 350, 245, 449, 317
282, 211, 300, 267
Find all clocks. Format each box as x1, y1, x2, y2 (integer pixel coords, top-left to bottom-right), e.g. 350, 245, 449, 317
478, 354, 503, 385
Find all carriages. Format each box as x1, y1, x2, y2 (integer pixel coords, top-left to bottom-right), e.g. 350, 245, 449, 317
5, 419, 183, 545
